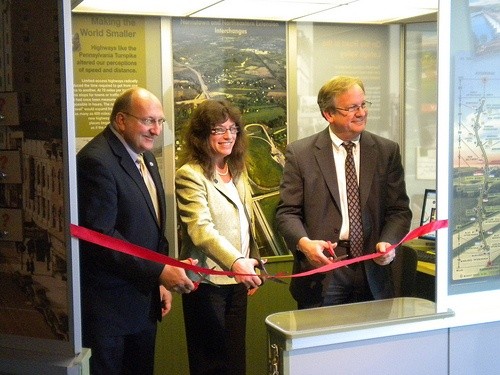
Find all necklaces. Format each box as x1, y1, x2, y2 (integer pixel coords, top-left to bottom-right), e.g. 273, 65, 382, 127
217, 167, 228, 175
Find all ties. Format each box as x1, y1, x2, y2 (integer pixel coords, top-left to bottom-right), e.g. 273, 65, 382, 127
136, 156, 160, 228
348, 142, 364, 262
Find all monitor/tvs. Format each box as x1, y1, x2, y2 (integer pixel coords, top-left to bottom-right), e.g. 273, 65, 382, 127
418, 189, 437, 241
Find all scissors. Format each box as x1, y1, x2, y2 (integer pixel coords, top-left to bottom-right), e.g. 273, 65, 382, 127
253, 257, 289, 287
327, 240, 349, 269
187, 258, 220, 291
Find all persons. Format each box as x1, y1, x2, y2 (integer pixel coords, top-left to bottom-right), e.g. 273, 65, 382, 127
175, 100, 267, 375
274, 77, 412, 309
77, 87, 199, 375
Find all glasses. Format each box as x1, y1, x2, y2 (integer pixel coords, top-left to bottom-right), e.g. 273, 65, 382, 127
336, 100, 372, 111
122, 111, 164, 125
210, 126, 240, 134
